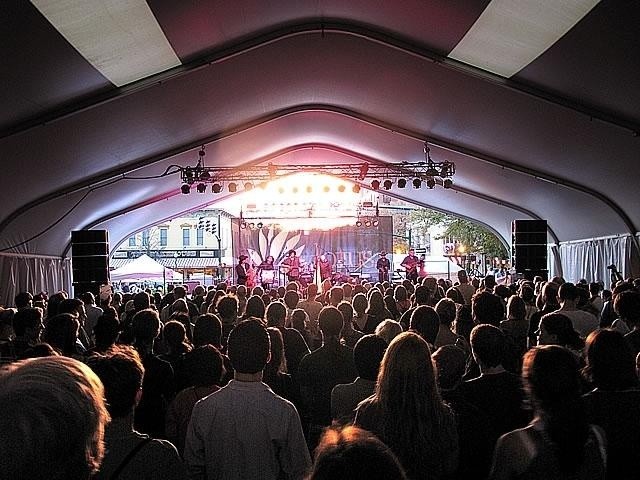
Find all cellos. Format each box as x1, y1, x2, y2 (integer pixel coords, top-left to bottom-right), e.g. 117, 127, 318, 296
244, 250, 256, 289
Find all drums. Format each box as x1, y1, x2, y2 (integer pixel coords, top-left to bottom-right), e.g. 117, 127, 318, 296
299, 273, 313, 288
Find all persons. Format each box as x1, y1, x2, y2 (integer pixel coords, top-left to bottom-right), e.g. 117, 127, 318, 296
282, 250, 304, 282
376, 252, 390, 282
1, 271, 640, 479
258, 256, 275, 289
235, 256, 251, 285
401, 248, 419, 285
314, 255, 331, 282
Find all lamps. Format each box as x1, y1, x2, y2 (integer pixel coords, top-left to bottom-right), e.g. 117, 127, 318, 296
356, 214, 380, 227
182, 143, 455, 193
241, 217, 264, 229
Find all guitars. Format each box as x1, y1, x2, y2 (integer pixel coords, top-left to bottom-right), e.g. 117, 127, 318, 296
279, 264, 307, 274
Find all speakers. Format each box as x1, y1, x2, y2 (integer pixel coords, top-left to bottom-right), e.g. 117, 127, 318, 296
511, 220, 547, 274
71, 230, 110, 283
